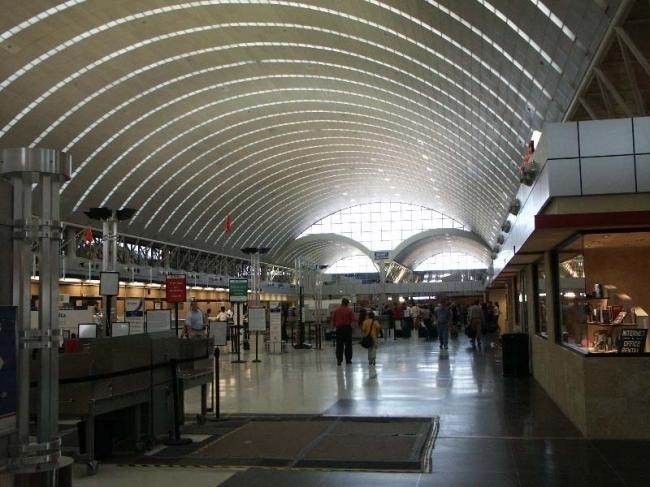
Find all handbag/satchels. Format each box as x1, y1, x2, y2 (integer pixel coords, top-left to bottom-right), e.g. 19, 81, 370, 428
361, 334, 374, 348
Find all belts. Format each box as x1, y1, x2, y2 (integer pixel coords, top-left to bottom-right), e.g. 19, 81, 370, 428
193, 328, 203, 331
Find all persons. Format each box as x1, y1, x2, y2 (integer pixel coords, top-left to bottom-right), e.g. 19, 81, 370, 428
87, 300, 98, 314
386, 296, 503, 354
362, 310, 381, 364
331, 297, 355, 364
183, 301, 211, 338
217, 305, 229, 322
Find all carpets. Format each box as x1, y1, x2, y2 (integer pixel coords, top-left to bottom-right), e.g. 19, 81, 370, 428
138, 410, 440, 474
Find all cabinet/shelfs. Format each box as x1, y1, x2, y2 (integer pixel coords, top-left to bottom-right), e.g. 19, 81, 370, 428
583, 296, 637, 326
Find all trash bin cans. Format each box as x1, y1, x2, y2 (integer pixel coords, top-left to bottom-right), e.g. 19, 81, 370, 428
394, 319, 411, 340
501, 334, 530, 377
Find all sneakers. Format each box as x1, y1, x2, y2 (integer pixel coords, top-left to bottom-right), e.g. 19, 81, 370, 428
369, 359, 375, 366
470, 340, 481, 345
440, 345, 448, 349
337, 360, 352, 365
